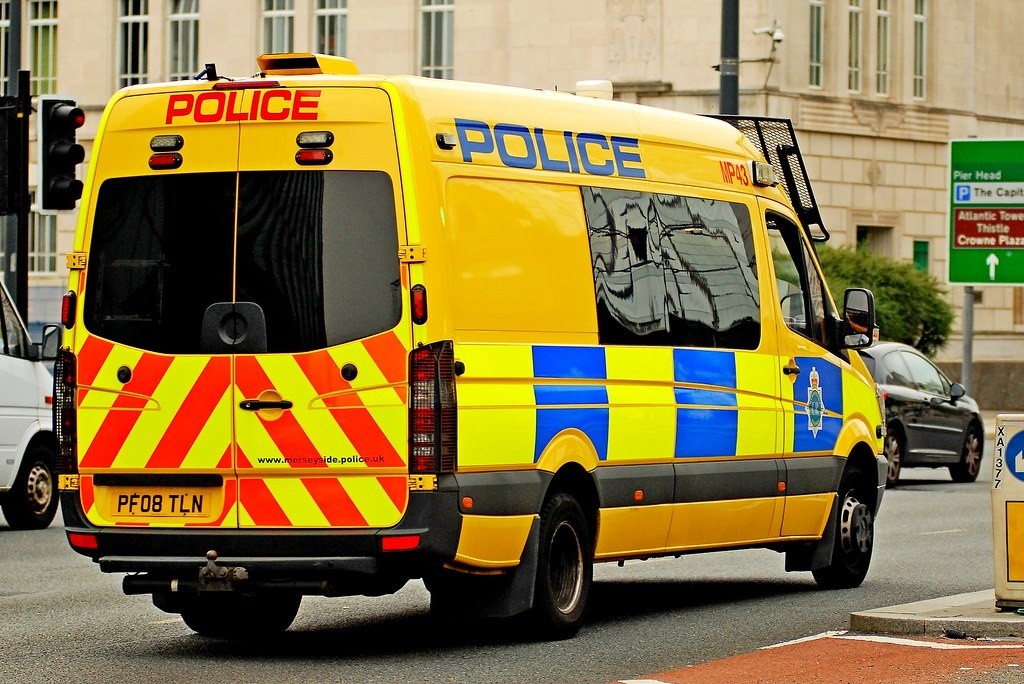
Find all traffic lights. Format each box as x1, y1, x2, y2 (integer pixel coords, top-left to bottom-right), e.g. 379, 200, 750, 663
36, 95, 85, 215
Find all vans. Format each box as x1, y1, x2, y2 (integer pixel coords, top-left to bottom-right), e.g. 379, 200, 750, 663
0, 276, 61, 531
53, 53, 888, 640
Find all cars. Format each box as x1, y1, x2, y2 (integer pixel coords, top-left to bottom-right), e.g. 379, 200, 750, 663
857, 343, 984, 488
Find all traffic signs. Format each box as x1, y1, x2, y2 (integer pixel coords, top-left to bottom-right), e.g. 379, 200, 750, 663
947, 138, 1024, 286
